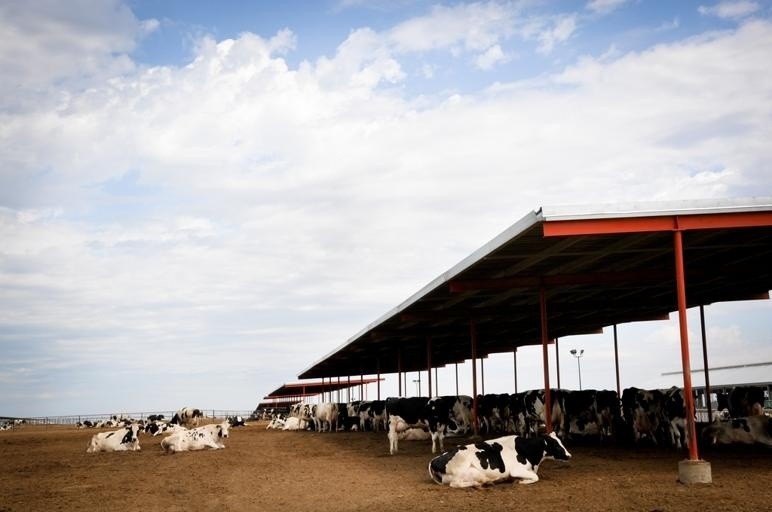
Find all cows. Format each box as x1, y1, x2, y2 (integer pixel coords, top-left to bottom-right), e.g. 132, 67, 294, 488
75, 406, 249, 437
160, 422, 232, 454
85, 422, 144, 454
427, 427, 573, 491
0, 419, 26, 431
253, 385, 771, 455
